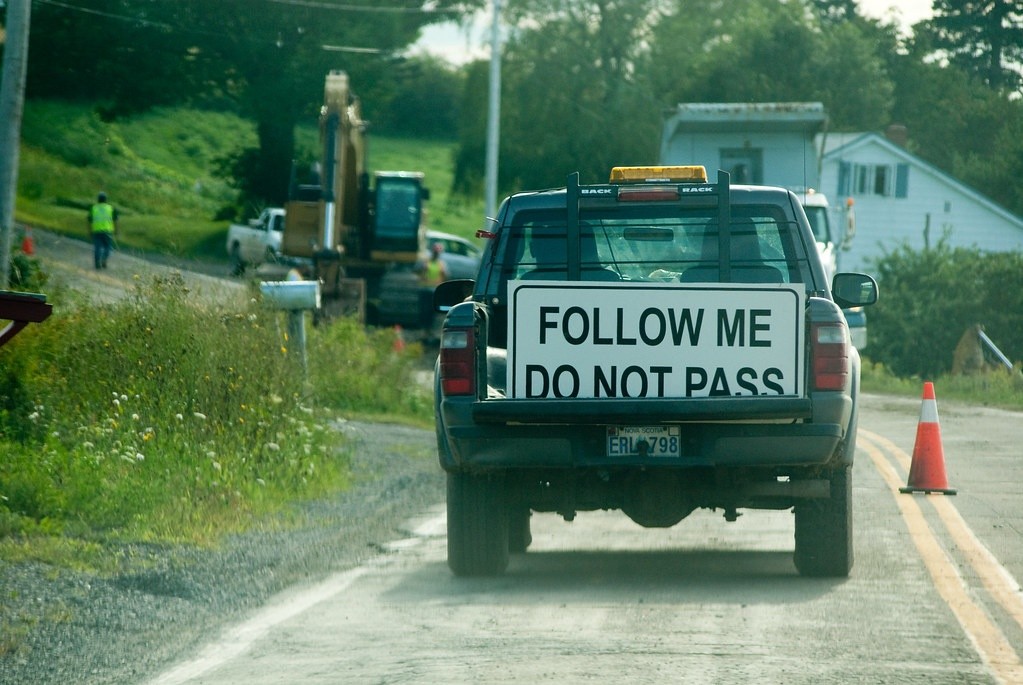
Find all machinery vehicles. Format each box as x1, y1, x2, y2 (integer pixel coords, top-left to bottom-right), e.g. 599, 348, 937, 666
280, 70, 434, 333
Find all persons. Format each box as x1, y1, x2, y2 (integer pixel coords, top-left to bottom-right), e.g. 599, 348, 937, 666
88, 193, 119, 269
520, 221, 551, 279
421, 242, 447, 336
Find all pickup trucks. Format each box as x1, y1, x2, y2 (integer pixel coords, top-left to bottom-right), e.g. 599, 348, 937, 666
228, 208, 294, 278
428, 163, 880, 582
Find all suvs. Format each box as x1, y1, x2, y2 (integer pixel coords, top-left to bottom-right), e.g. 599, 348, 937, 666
514, 186, 868, 355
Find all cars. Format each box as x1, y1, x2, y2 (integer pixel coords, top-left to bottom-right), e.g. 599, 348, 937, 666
366, 233, 484, 329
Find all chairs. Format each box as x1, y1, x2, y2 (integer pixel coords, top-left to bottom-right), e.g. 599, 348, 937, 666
522, 220, 621, 281
681, 215, 783, 283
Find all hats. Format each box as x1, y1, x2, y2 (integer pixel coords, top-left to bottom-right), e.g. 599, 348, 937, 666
433, 243, 442, 252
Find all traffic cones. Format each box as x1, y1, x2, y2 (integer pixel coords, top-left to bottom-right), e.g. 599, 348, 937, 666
896, 380, 959, 496
18, 225, 38, 254
